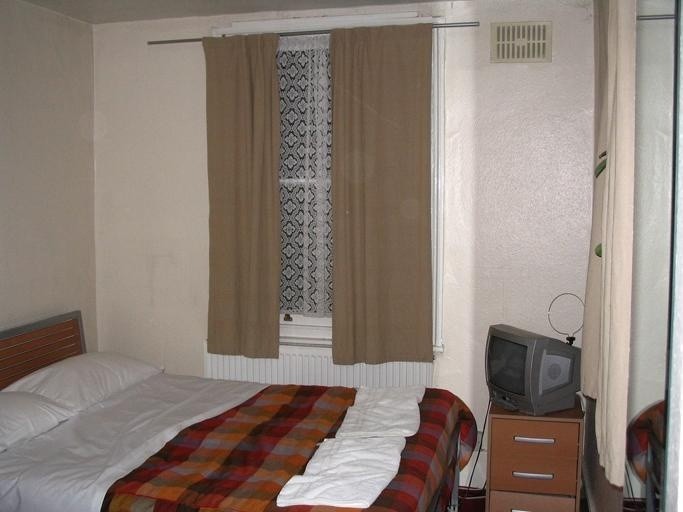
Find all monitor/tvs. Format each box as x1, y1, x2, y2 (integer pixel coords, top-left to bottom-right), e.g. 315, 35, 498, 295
485, 324, 581, 416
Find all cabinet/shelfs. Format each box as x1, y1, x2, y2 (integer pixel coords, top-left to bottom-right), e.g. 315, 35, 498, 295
484, 391, 583, 512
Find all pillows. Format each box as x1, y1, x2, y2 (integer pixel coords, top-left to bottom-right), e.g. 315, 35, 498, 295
0, 390, 78, 453
3, 349, 164, 413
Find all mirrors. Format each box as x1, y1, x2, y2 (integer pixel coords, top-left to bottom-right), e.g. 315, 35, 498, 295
622, 0, 679, 512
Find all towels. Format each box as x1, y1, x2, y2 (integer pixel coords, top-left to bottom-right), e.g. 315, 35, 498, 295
335, 384, 425, 437
276, 435, 406, 508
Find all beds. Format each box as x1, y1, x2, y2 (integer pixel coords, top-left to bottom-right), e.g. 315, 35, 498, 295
623, 401, 666, 512
0, 309, 478, 512
